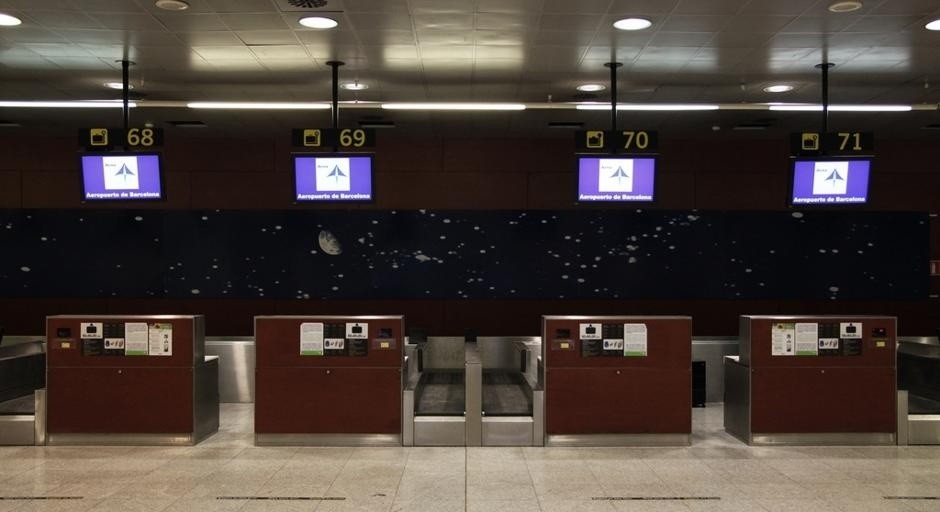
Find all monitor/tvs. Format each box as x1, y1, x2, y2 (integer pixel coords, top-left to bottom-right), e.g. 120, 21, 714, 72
785, 155, 874, 207
76, 150, 167, 205
574, 151, 658, 207
289, 150, 377, 204
